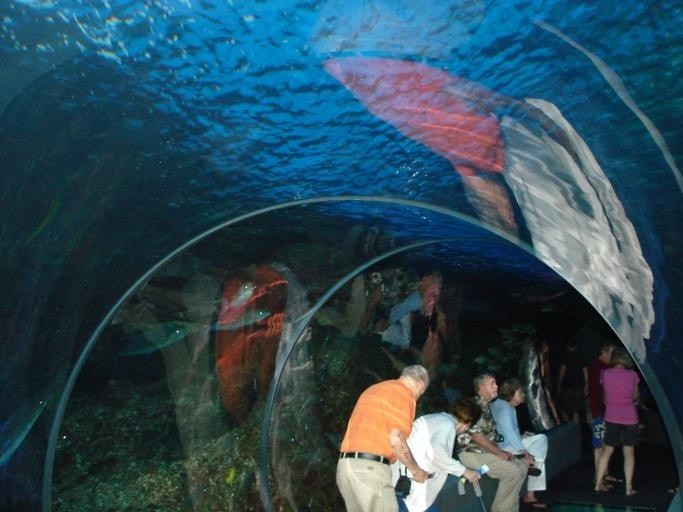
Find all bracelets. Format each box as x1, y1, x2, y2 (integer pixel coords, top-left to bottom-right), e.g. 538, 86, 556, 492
411, 466, 420, 476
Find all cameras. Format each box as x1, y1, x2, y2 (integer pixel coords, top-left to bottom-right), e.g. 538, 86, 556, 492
495, 434, 504, 442
394, 476, 411, 498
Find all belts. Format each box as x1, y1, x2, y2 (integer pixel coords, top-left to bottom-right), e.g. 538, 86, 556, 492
338, 450, 391, 467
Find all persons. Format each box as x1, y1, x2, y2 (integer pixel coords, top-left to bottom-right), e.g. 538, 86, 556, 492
387, 399, 483, 512
587, 334, 623, 490
486, 377, 549, 512
335, 364, 432, 512
305, 235, 461, 418
452, 371, 531, 512
539, 334, 562, 425
593, 346, 642, 497
553, 336, 590, 426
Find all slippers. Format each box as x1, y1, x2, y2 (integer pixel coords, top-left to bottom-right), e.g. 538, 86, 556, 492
523, 500, 548, 508
592, 473, 639, 498
